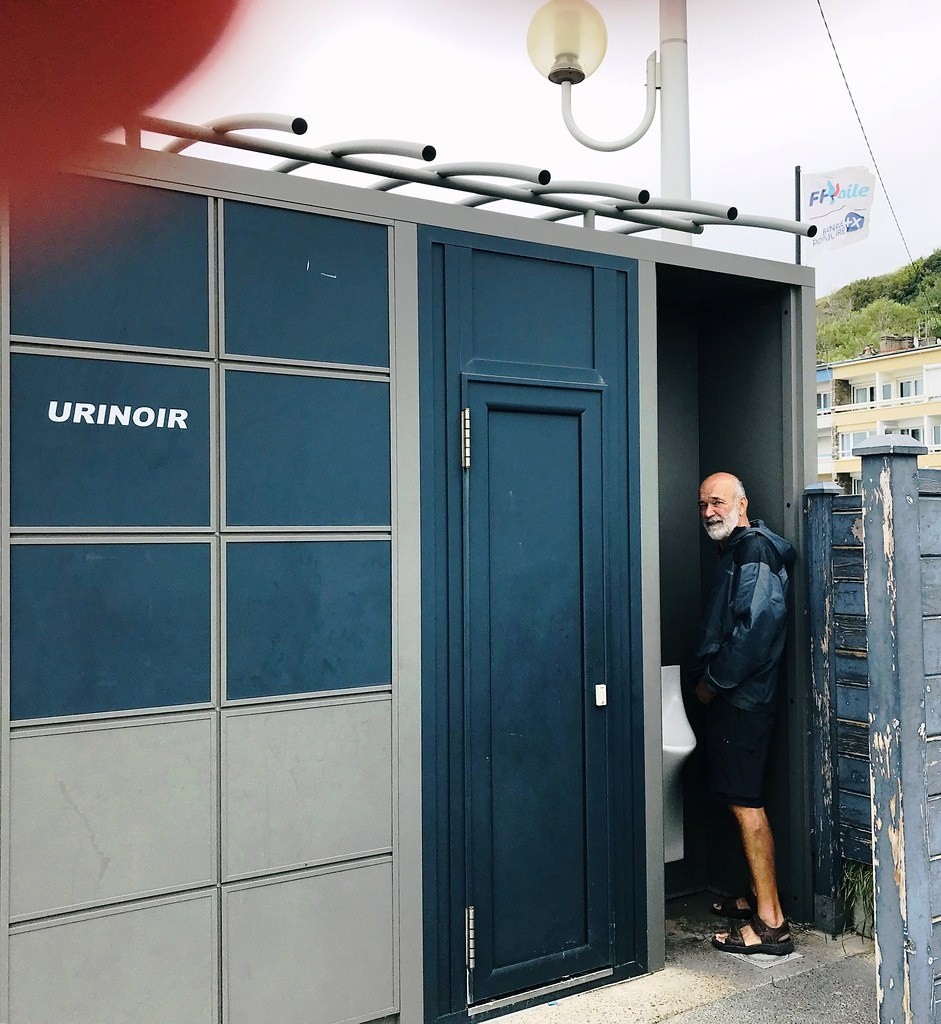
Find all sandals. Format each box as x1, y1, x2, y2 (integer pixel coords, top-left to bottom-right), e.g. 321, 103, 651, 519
712, 917, 794, 955
710, 891, 758, 919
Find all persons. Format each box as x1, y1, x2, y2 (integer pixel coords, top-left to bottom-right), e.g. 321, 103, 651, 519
694, 473, 800, 956
